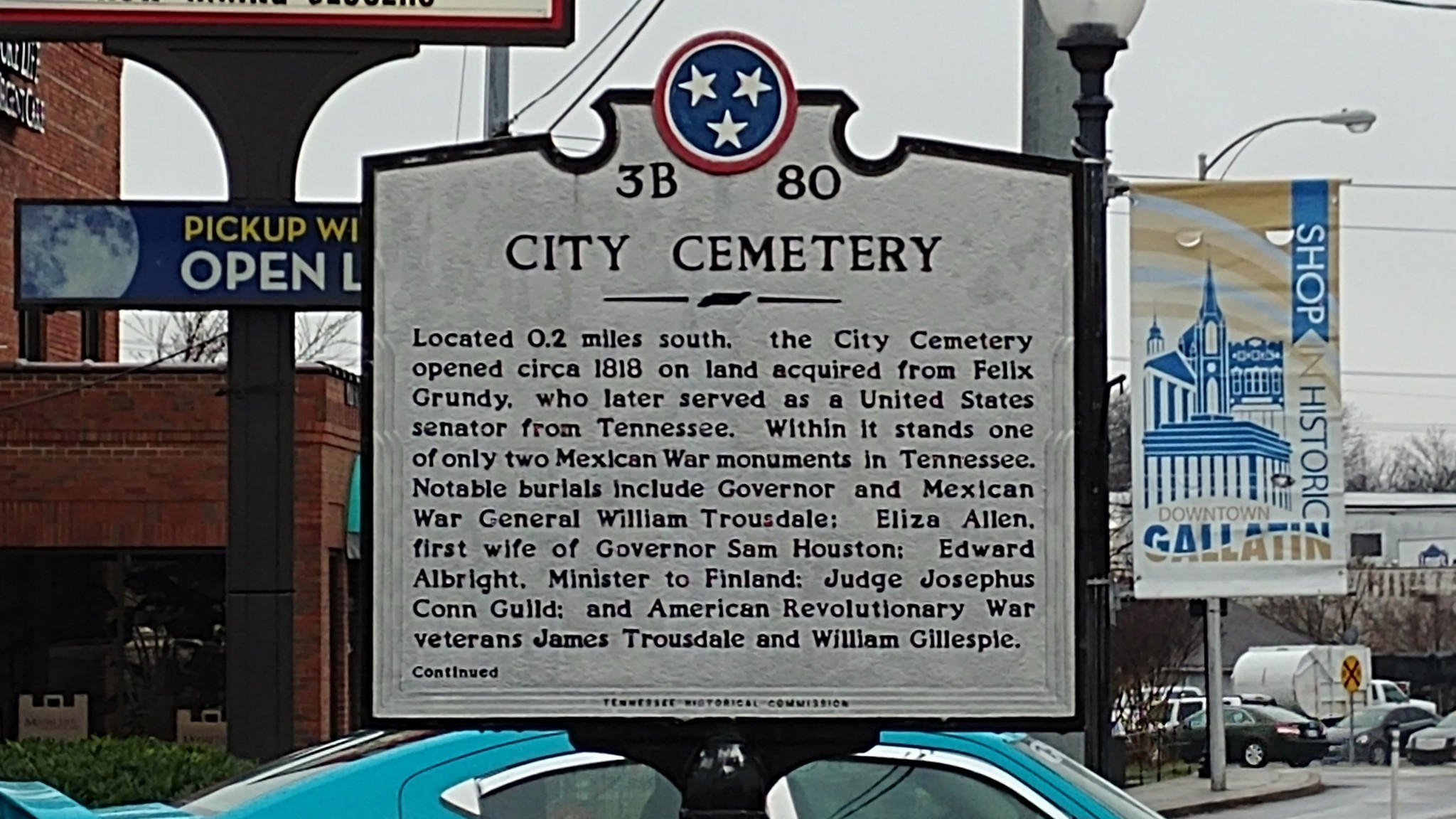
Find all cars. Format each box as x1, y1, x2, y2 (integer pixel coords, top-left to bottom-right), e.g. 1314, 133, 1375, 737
1120, 685, 1331, 769
1326, 700, 1456, 767
0, 711, 1169, 819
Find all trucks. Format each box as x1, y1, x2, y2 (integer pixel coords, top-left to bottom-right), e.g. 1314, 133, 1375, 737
1229, 643, 1408, 724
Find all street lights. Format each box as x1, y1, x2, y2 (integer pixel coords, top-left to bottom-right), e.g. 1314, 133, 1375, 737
1198, 106, 1377, 792
1036, 0, 1147, 775
